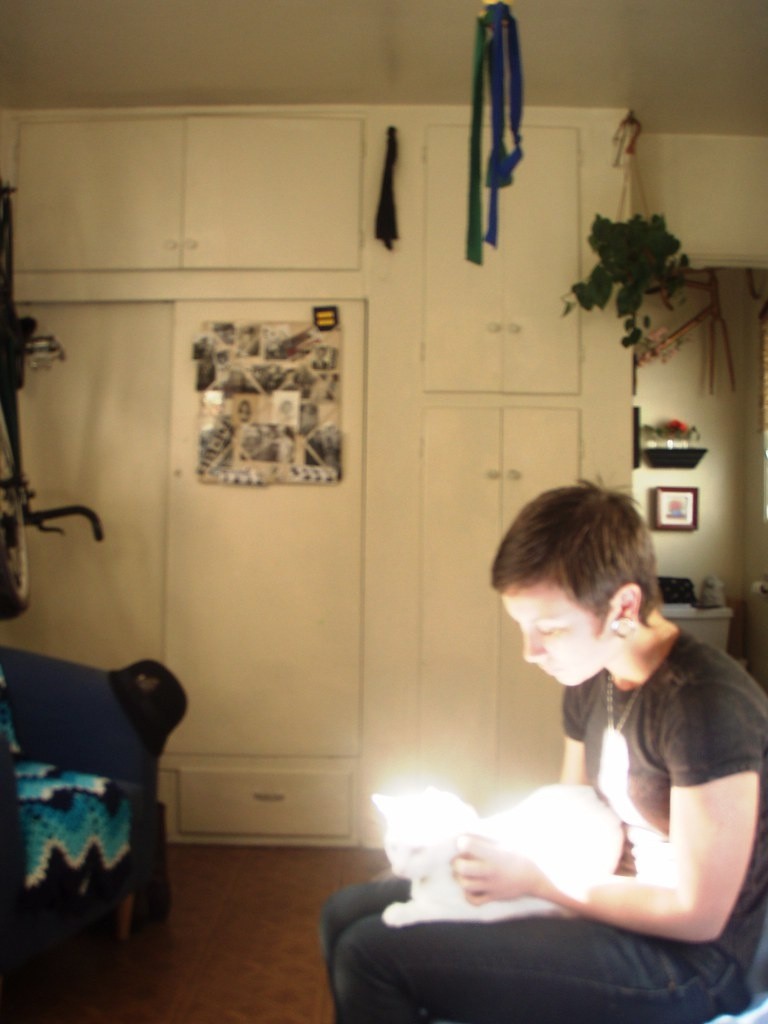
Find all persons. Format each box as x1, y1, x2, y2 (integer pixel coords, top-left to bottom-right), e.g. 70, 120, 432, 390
319, 480, 768, 1024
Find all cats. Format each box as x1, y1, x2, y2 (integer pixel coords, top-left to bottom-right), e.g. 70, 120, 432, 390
371, 785, 624, 928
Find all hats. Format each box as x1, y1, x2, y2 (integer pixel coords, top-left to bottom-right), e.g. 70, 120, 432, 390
107, 658, 187, 760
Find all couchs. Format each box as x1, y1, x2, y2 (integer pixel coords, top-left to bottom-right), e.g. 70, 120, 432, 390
1, 646, 189, 1023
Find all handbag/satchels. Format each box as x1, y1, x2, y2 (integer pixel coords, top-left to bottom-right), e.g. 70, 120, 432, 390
657, 576, 697, 603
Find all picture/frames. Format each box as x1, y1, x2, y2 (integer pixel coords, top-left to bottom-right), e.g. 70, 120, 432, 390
657, 485, 698, 531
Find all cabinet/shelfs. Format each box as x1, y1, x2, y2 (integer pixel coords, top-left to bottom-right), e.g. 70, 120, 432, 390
660, 602, 733, 653
13, 299, 364, 827
424, 121, 585, 812
9, 119, 370, 274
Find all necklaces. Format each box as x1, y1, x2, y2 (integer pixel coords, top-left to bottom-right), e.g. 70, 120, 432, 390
607, 675, 641, 735
